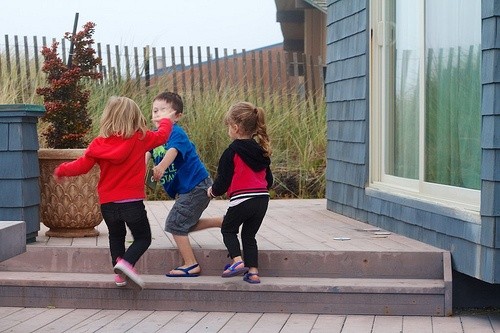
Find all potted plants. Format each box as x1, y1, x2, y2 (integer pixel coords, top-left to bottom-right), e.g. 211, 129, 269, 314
35, 22, 103, 238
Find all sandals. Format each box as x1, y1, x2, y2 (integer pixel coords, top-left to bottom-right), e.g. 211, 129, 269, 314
243, 273, 260, 283
166, 263, 199, 277
222, 261, 249, 277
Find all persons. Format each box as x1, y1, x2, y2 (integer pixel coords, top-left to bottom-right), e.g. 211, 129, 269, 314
145, 91, 214, 277
52, 96, 177, 292
205, 102, 274, 284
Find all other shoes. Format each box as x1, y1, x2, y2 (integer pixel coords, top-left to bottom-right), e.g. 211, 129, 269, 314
114, 259, 144, 291
114, 274, 126, 286
